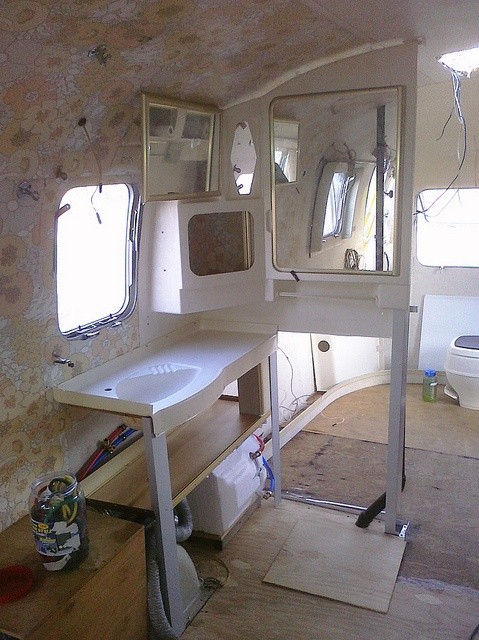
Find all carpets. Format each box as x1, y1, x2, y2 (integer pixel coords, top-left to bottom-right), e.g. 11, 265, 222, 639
282, 383, 479, 459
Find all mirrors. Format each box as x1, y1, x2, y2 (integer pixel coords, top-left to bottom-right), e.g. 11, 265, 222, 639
267, 84, 405, 276
140, 92, 224, 204
273, 118, 302, 188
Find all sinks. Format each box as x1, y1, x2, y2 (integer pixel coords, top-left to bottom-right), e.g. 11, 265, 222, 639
115, 363, 200, 405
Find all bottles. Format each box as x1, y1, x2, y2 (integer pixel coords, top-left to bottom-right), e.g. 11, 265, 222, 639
421, 369, 438, 402
28, 470, 90, 572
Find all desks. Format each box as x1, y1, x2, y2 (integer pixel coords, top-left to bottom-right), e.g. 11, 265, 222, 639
0, 505, 149, 640
